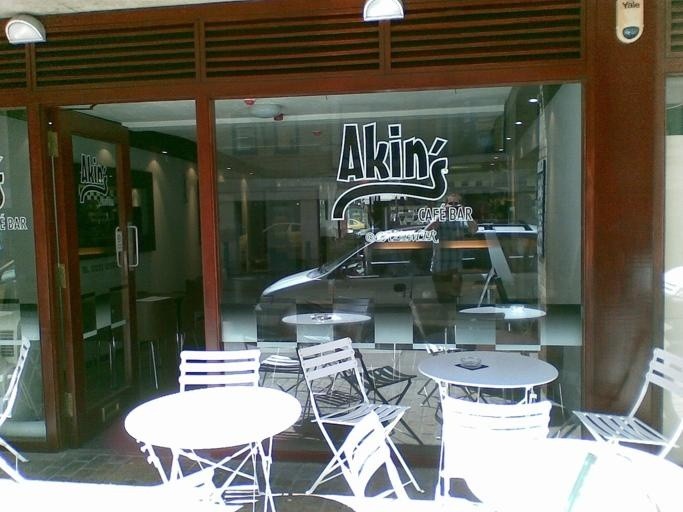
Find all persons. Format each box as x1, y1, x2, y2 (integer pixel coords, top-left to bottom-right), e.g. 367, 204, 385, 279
424, 192, 477, 304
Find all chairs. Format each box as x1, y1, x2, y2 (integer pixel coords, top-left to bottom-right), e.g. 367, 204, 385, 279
436, 395, 552, 503
170, 350, 277, 511
0, 334, 31, 481
296, 338, 423, 508
552, 348, 683, 458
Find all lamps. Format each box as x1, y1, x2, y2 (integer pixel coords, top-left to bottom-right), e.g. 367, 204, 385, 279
361, 0, 404, 22
5, 13, 48, 44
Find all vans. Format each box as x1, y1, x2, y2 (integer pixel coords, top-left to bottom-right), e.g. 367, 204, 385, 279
256, 222, 538, 340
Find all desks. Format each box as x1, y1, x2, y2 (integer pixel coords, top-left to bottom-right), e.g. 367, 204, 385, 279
281, 313, 371, 326
460, 306, 545, 322
416, 349, 559, 404
466, 438, 683, 509
124, 387, 302, 512
